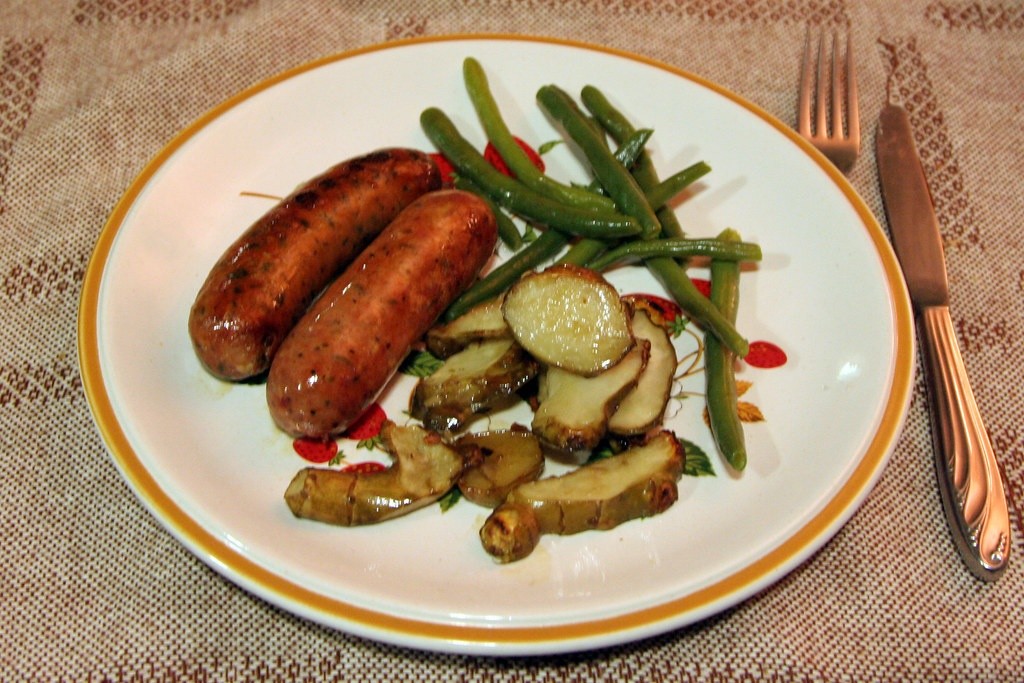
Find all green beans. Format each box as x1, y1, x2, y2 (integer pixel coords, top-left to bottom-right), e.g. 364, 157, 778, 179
419, 55, 764, 470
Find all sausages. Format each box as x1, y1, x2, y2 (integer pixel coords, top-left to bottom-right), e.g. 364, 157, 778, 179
267, 189, 500, 441
188, 145, 442, 381
